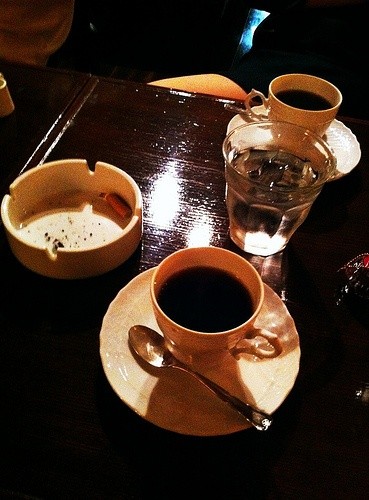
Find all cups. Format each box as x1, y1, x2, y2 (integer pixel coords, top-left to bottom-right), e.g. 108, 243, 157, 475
150, 246, 282, 360
245, 73, 342, 141
221, 120, 337, 257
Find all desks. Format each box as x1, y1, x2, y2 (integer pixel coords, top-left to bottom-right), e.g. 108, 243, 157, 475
0, 56, 369, 500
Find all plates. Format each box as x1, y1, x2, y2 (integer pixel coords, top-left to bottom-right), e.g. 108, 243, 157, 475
99, 266, 301, 436
227, 103, 361, 183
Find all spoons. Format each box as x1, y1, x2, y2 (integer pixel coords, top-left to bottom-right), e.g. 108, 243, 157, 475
128, 324, 273, 431
